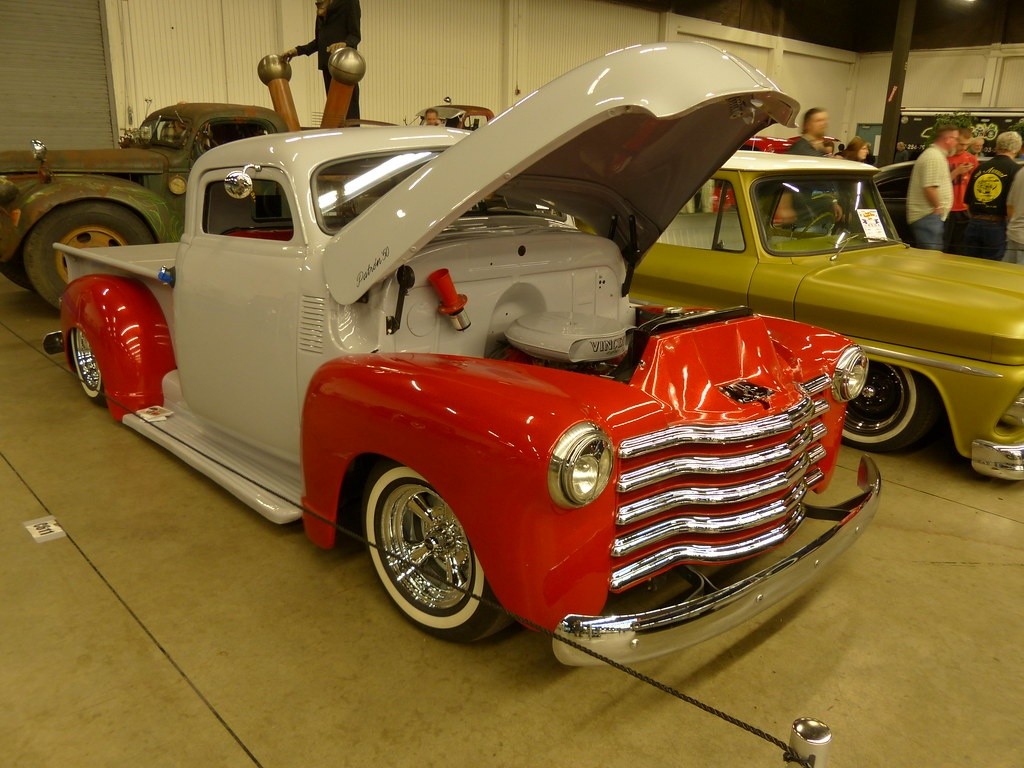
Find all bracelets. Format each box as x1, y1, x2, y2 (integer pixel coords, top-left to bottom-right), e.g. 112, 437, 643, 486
830, 199, 837, 206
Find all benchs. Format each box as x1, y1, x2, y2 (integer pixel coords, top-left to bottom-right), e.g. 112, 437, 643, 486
655, 211, 745, 253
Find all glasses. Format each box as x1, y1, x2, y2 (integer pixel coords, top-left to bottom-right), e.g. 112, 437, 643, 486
944, 136, 959, 141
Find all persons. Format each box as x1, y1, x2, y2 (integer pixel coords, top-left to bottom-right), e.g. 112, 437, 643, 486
424, 109, 442, 127
786, 106, 1024, 265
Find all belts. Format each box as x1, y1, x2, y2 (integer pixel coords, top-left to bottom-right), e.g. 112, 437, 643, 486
971, 213, 1004, 221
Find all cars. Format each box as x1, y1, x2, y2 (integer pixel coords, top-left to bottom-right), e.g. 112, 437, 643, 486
866, 156, 1024, 239
563, 148, 1023, 484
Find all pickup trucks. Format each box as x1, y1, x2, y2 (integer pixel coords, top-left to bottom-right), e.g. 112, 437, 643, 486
42, 36, 883, 668
0, 43, 838, 316
